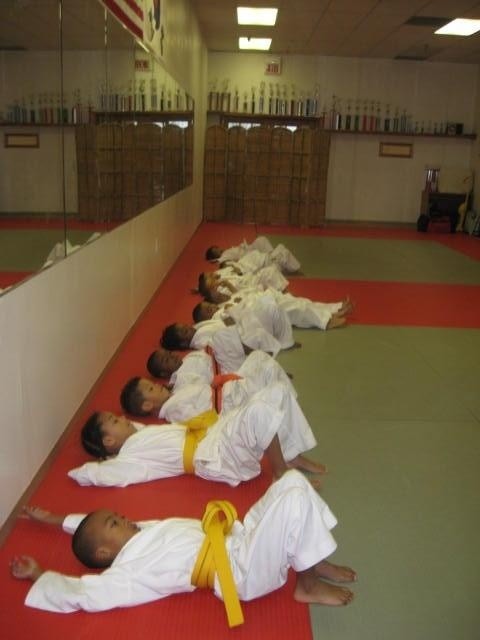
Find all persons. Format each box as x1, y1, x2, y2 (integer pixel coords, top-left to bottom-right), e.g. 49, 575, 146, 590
7, 467, 360, 628
120, 236, 353, 425
66, 380, 328, 494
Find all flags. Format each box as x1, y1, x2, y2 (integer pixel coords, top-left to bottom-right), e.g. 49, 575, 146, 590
100, 0, 145, 41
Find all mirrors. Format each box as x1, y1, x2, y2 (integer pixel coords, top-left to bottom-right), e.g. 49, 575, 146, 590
0, 2, 197, 295
